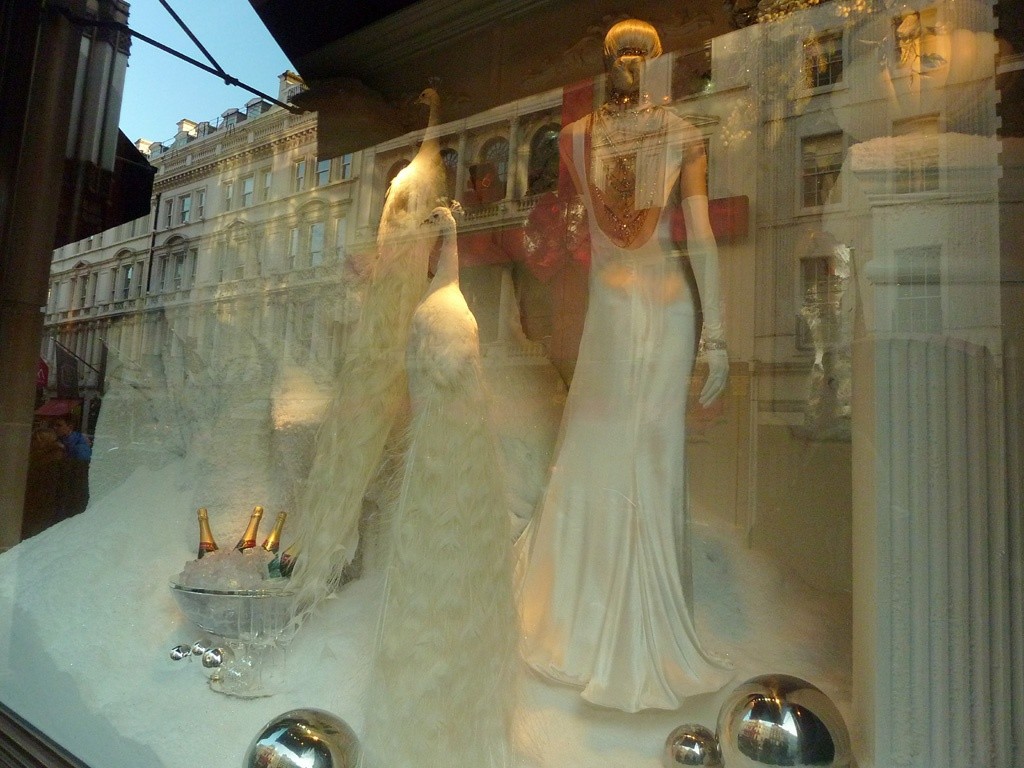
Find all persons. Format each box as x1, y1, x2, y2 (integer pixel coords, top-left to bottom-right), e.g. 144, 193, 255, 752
22, 415, 93, 535
506, 19, 737, 712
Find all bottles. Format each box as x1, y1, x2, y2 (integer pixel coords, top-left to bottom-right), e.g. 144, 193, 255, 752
234, 506, 264, 553
198, 508, 219, 559
261, 512, 288, 557
269, 534, 302, 579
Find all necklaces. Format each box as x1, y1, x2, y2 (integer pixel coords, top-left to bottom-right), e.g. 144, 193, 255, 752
589, 92, 666, 247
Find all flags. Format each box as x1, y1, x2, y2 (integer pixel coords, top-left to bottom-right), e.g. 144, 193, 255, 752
56, 344, 79, 401
37, 358, 50, 385
97, 342, 109, 393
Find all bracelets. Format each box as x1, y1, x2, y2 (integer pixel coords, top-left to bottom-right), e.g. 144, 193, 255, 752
704, 342, 727, 349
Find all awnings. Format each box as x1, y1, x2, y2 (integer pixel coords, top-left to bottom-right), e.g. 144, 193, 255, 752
55, 130, 154, 247
35, 400, 81, 417
249, 0, 789, 163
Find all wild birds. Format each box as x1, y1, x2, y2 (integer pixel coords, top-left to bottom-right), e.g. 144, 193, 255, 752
350, 197, 553, 768
285, 74, 450, 628
